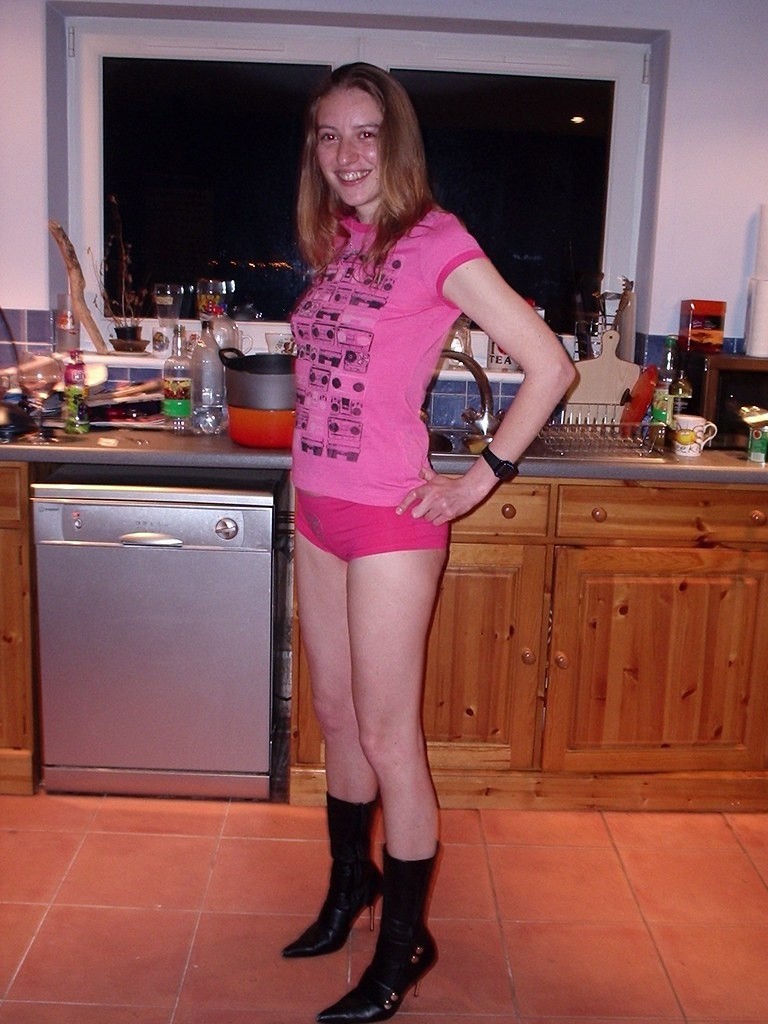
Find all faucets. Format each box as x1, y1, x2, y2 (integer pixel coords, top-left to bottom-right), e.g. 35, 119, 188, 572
439, 343, 506, 437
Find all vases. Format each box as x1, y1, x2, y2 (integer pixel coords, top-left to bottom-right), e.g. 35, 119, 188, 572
114, 326, 142, 340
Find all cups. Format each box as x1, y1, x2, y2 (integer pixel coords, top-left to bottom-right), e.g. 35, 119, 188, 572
195, 279, 222, 321
154, 282, 182, 327
238, 330, 253, 355
55, 294, 80, 356
673, 414, 718, 455
724, 337, 744, 354
264, 332, 299, 359
153, 327, 172, 358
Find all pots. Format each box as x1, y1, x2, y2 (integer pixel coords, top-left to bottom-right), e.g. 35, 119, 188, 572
219, 347, 298, 410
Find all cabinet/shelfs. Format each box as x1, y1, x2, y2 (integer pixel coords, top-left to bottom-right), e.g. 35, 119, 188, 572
286, 470, 768, 814
0, 461, 42, 797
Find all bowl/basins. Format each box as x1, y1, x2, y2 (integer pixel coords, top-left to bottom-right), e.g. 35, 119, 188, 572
226, 405, 297, 448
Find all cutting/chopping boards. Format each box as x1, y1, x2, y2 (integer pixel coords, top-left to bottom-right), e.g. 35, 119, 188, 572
563, 330, 641, 433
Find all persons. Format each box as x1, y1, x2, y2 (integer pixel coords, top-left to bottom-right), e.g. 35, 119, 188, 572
283, 59, 580, 1024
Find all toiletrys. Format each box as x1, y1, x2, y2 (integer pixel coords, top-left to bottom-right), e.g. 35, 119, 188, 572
448, 312, 474, 370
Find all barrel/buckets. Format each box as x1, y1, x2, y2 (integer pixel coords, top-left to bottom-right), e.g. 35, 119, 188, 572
485, 304, 546, 372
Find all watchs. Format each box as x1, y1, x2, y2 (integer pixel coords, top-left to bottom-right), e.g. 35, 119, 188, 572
481, 445, 519, 481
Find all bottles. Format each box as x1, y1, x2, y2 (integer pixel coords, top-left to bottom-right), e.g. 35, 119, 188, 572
668, 370, 693, 452
650, 335, 683, 447
186, 335, 199, 358
190, 321, 225, 434
163, 324, 192, 429
64, 349, 90, 435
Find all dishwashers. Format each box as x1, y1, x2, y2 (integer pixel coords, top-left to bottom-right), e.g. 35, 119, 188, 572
27, 482, 275, 802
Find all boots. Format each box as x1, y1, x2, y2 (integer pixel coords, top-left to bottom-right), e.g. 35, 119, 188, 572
282, 791, 386, 957
316, 843, 439, 1024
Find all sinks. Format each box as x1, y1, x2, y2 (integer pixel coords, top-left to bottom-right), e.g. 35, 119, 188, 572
429, 429, 466, 455
